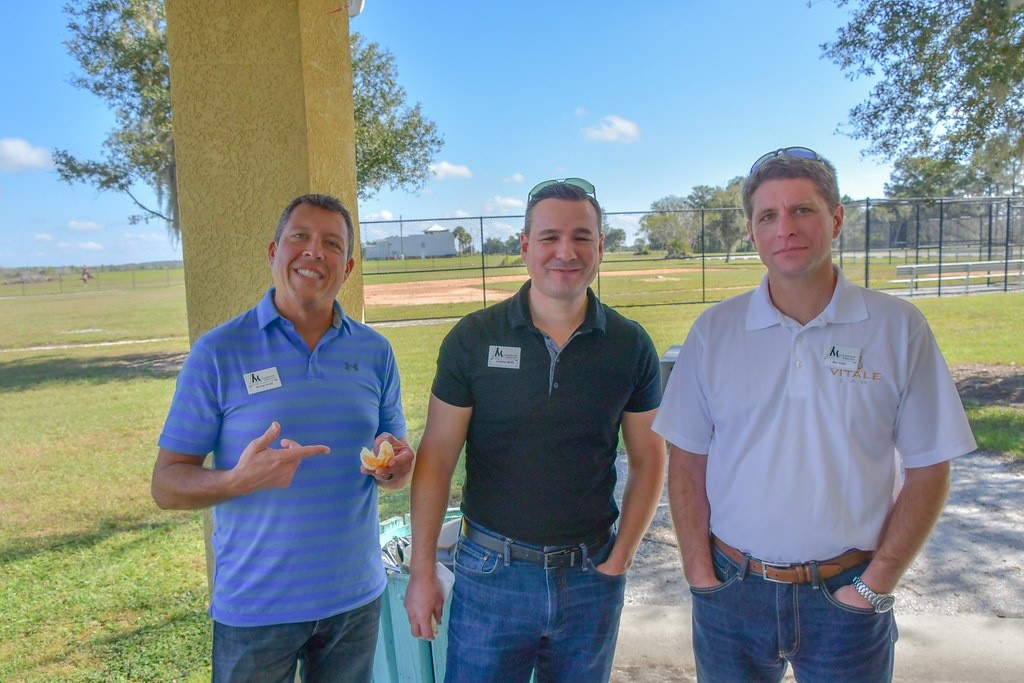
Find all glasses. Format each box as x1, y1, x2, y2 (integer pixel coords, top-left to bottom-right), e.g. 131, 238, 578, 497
750, 146, 824, 175
527, 178, 596, 203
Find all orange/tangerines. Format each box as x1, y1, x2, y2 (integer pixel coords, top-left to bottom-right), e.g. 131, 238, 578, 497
360, 441, 395, 470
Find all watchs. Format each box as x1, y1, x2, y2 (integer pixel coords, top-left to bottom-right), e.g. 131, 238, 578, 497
853, 577, 896, 614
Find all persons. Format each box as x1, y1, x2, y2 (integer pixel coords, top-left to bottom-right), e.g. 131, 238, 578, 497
150, 192, 415, 683
650, 146, 980, 683
403, 177, 665, 683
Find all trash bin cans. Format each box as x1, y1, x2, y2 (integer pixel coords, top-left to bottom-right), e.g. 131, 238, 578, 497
368, 505, 536, 683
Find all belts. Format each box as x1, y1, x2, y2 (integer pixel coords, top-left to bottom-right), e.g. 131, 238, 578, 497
461, 516, 614, 570
710, 532, 874, 584
